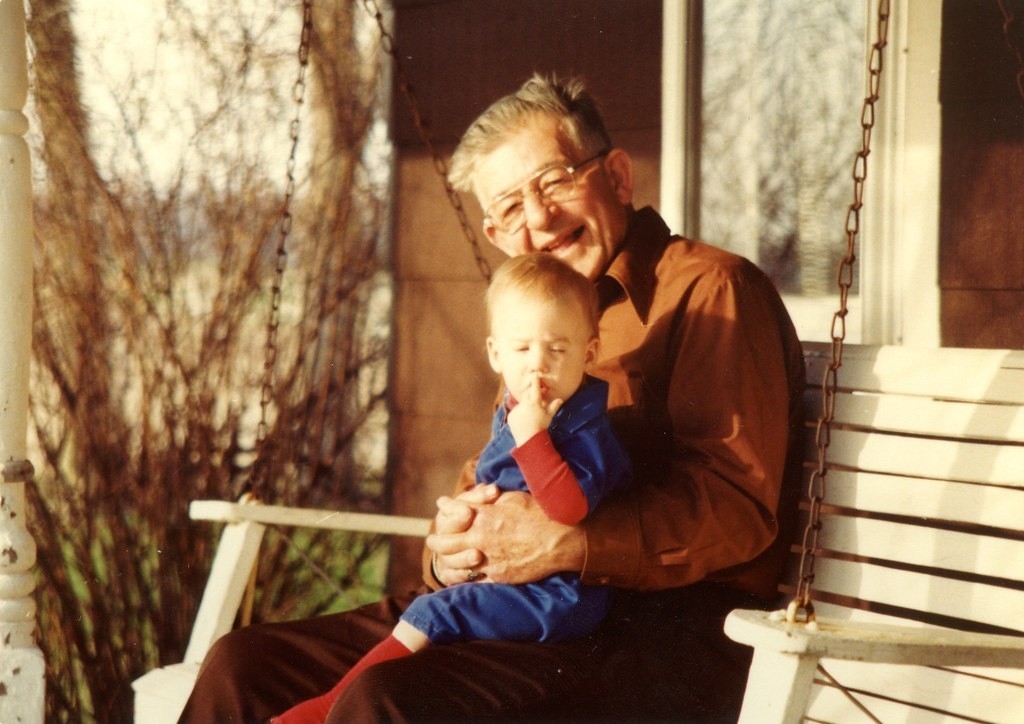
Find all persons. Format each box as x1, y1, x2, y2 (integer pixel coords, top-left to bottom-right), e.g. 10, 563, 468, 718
269, 252, 630, 724
178, 71, 810, 724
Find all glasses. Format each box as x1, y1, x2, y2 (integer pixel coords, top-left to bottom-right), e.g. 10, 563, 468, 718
486, 149, 607, 234
